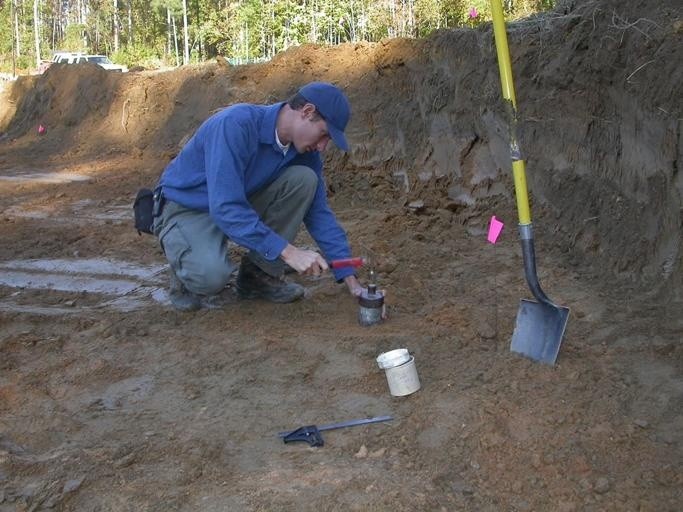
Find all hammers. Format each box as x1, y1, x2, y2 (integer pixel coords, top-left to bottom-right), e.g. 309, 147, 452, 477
282, 253, 379, 275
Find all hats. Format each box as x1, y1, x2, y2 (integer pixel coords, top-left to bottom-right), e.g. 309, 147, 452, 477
297, 79, 352, 154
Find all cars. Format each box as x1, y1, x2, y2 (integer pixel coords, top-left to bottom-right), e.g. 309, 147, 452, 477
40, 52, 128, 76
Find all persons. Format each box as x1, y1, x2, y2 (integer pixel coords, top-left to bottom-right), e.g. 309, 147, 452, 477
149, 82, 388, 322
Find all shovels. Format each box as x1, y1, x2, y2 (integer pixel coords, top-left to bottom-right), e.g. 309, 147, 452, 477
489, 0, 570, 367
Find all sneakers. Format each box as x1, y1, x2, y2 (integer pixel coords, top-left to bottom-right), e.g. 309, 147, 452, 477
167, 266, 201, 313
234, 255, 305, 305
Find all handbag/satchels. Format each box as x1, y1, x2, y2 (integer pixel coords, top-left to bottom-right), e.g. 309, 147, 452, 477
131, 187, 159, 235
